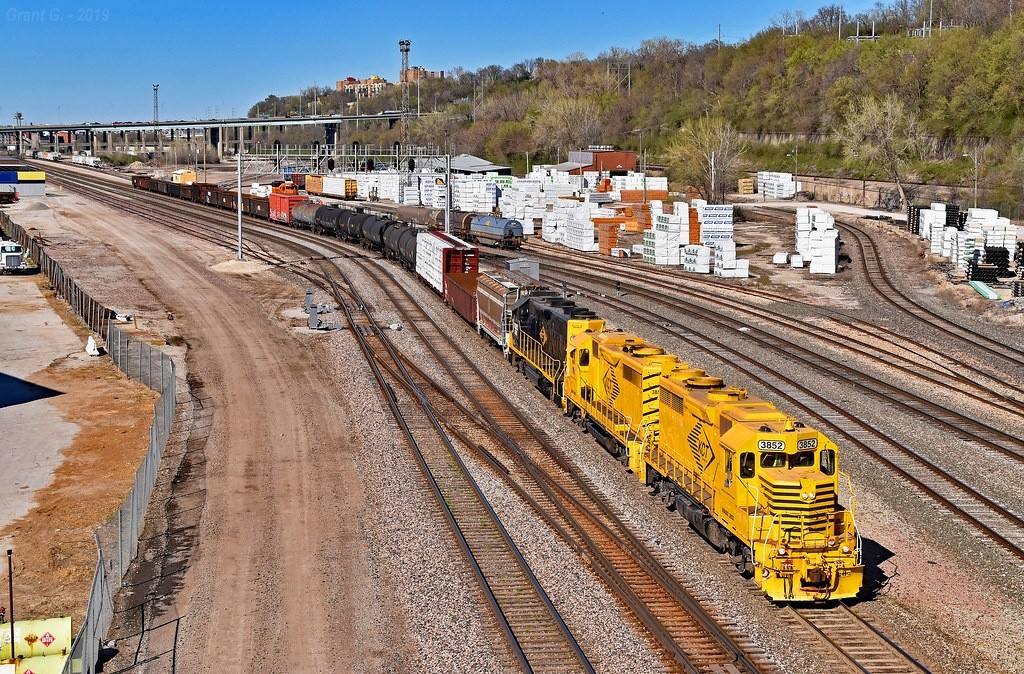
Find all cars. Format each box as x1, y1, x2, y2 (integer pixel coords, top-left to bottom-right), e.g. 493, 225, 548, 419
82, 119, 218, 125
298, 190, 309, 195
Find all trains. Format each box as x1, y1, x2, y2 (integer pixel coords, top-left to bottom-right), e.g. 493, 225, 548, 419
290, 173, 358, 200
329, 200, 523, 251
131, 171, 865, 604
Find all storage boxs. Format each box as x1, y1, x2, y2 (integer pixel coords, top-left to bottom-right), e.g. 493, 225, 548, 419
250, 165, 1020, 292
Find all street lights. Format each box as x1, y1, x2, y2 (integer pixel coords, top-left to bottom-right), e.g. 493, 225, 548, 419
442, 129, 450, 172
18, 113, 24, 159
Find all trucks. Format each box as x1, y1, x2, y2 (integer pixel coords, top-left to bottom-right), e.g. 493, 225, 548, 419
25, 150, 59, 162
0, 239, 27, 275
72, 155, 101, 167
